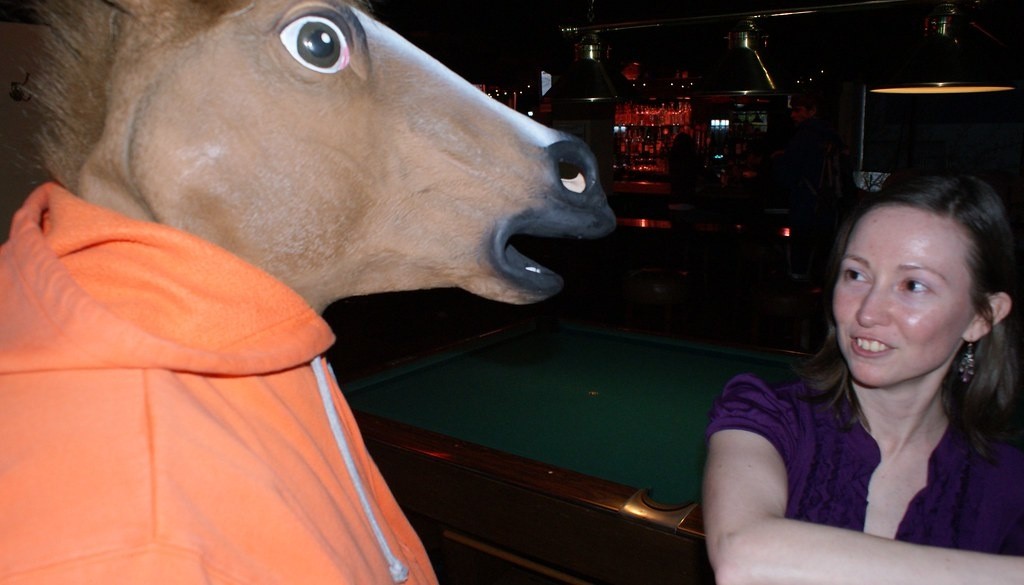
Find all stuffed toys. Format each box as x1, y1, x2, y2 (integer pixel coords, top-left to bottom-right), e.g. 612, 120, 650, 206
0, 0, 619, 585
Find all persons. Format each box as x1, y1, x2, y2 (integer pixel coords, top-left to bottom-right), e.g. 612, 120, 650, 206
699, 176, 1024, 585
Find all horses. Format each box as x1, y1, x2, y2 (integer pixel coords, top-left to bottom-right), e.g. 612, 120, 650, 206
0, 2, 618, 316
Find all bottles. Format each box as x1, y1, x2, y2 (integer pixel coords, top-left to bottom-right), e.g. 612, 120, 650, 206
613, 95, 748, 183
